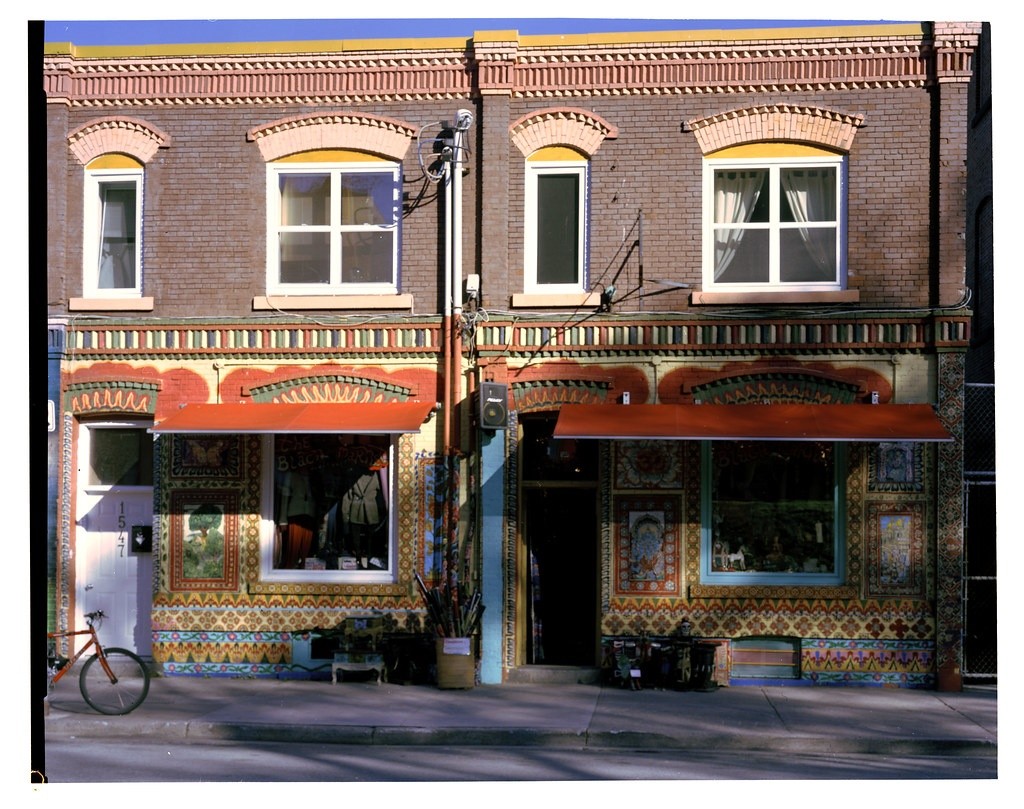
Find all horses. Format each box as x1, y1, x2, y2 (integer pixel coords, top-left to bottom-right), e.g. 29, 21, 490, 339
726, 545, 746, 571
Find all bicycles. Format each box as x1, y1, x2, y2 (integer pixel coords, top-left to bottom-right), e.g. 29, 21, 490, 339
47, 609, 150, 716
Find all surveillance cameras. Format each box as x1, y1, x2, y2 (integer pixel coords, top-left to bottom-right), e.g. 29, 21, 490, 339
466, 273, 479, 293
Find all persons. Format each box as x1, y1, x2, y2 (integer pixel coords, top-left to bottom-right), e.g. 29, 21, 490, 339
279, 469, 319, 569
341, 468, 381, 565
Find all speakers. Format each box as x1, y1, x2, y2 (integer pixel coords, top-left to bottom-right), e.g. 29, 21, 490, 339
474, 382, 509, 430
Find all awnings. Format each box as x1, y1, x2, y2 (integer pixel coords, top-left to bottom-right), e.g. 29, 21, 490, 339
554, 403, 954, 442
144, 400, 438, 433
